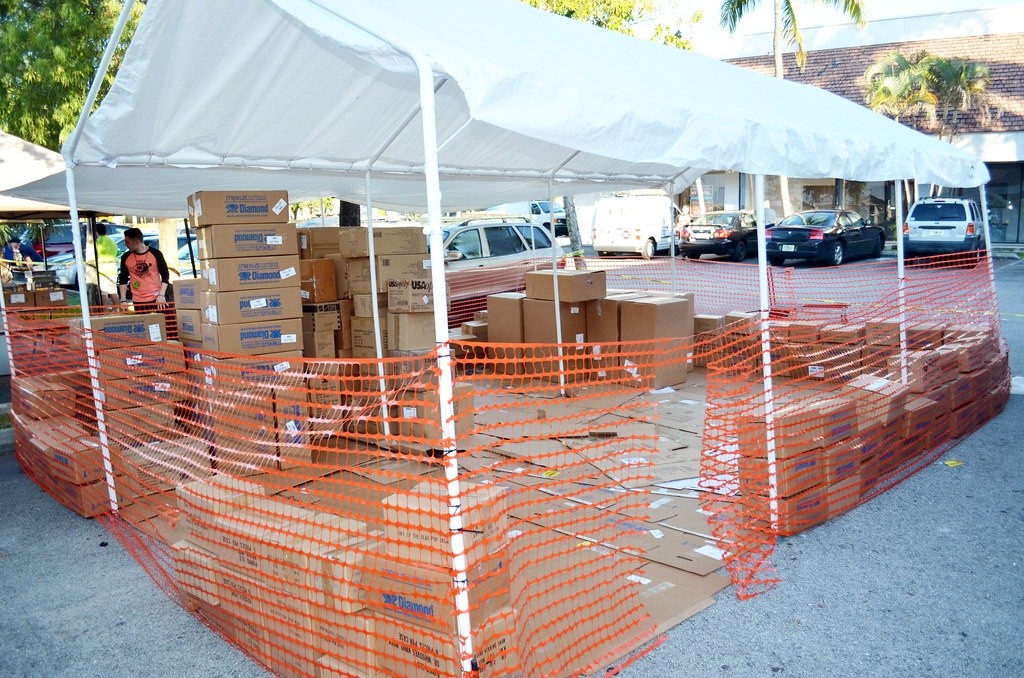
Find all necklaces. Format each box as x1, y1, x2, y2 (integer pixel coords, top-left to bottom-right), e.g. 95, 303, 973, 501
132, 245, 147, 289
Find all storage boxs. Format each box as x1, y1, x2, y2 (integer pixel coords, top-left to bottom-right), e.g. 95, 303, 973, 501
3, 190, 1008, 678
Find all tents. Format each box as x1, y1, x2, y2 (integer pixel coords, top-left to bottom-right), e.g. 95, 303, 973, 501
0, 0, 1005, 674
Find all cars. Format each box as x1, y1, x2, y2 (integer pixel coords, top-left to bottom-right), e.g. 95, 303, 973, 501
678, 208, 775, 262
293, 210, 421, 227
765, 209, 888, 266
8, 223, 201, 299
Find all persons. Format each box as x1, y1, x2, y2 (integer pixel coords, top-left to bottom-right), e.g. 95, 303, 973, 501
85, 223, 119, 316
5, 236, 43, 281
118, 228, 169, 312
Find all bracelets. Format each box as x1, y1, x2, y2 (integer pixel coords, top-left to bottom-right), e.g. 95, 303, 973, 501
120, 300, 127, 303
159, 295, 165, 296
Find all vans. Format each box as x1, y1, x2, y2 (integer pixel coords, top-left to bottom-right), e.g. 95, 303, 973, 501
426, 213, 569, 303
591, 193, 691, 260
489, 199, 568, 238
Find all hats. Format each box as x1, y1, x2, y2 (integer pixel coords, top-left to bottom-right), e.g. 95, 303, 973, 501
8, 237, 21, 242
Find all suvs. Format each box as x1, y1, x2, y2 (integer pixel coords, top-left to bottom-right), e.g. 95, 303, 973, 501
901, 195, 992, 270
